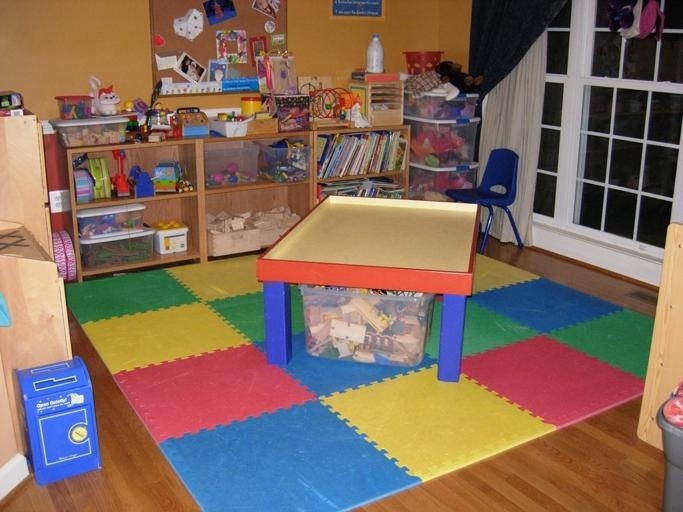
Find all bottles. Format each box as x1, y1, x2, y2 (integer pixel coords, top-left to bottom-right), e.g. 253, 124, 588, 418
366, 33, 384, 73
150, 102, 167, 127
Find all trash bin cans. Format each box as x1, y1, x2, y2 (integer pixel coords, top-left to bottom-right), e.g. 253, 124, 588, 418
657, 401, 683, 511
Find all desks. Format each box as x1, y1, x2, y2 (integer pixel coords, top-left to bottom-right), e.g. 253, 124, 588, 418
257, 195, 480, 381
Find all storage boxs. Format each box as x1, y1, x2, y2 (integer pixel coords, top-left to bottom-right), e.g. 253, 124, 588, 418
151, 221, 189, 254
209, 227, 260, 257
403, 115, 480, 169
403, 90, 480, 119
76, 204, 148, 241
77, 229, 156, 270
202, 141, 260, 187
298, 285, 436, 368
248, 223, 293, 248
409, 161, 481, 202
255, 138, 313, 183
50, 118, 129, 149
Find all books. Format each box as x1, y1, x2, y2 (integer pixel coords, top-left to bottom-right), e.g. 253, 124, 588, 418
315, 129, 405, 199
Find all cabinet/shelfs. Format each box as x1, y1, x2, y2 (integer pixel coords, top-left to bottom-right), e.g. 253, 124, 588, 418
0, 107, 54, 262
0, 220, 73, 458
636, 223, 683, 453
57, 124, 411, 283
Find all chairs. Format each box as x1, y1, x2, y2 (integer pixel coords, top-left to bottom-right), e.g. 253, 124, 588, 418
446, 149, 523, 255
